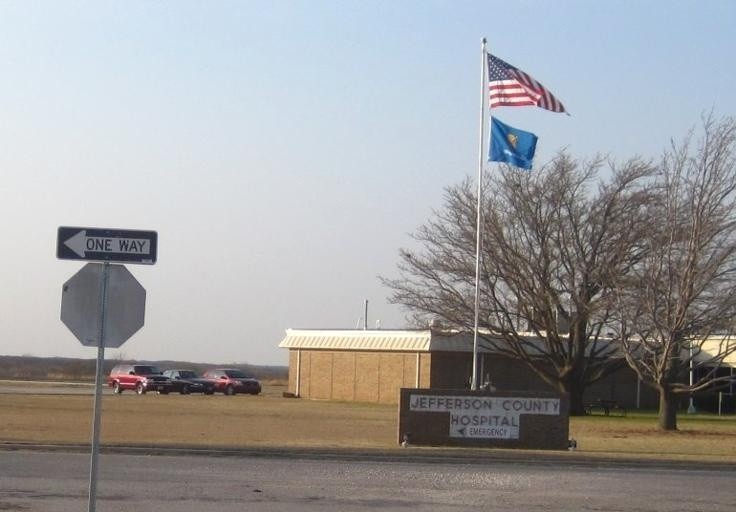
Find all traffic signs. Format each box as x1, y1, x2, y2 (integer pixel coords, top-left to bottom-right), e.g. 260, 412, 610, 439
57, 225, 157, 264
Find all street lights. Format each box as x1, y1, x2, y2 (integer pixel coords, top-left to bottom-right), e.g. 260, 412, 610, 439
687, 324, 697, 413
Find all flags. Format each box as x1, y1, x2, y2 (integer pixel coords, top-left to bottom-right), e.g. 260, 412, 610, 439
485, 51, 571, 173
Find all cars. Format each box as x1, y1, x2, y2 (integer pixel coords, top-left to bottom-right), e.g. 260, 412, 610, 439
108, 363, 261, 396
